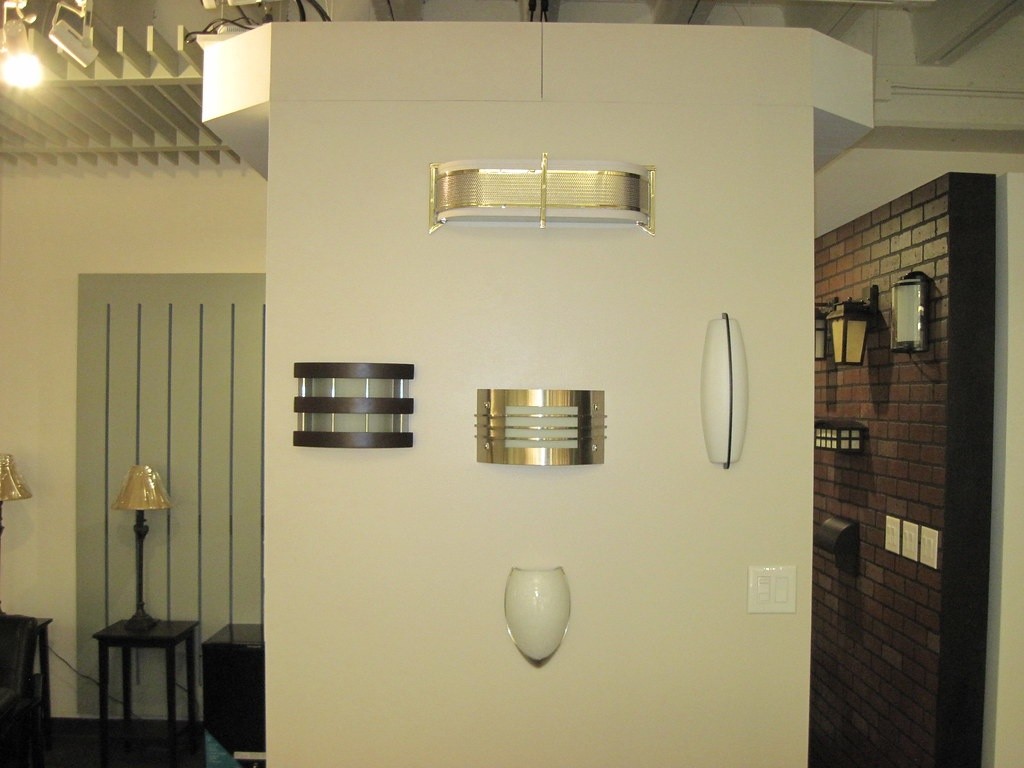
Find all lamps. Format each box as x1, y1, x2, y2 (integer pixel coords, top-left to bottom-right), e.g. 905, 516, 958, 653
474, 389, 607, 467
0, 453, 32, 627
111, 464, 174, 630
814, 418, 867, 455
888, 272, 933, 352
504, 567, 571, 668
429, 155, 655, 237
0, 19, 42, 89
815, 298, 871, 368
291, 362, 414, 448
700, 313, 750, 467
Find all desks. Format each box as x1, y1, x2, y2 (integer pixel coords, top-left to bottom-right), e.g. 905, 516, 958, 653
0, 617, 52, 768
202, 624, 264, 768
92, 619, 200, 768
